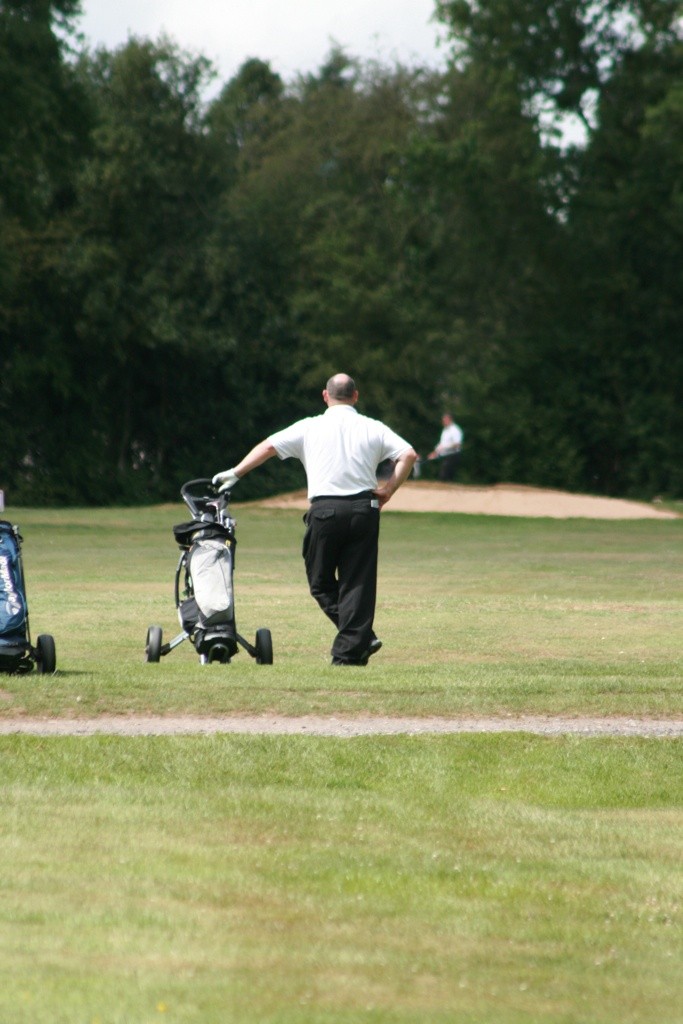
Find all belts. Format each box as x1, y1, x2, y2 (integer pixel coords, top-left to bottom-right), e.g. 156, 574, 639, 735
312, 491, 375, 502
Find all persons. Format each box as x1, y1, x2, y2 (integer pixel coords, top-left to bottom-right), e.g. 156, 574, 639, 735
211, 372, 415, 666
427, 411, 464, 482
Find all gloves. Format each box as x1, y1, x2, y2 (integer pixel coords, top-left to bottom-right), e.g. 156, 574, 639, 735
212, 468, 242, 493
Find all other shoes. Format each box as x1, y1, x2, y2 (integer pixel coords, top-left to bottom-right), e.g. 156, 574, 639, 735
369, 632, 382, 655
331, 656, 348, 665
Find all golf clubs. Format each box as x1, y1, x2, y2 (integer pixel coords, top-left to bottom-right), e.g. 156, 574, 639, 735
200, 498, 238, 532
